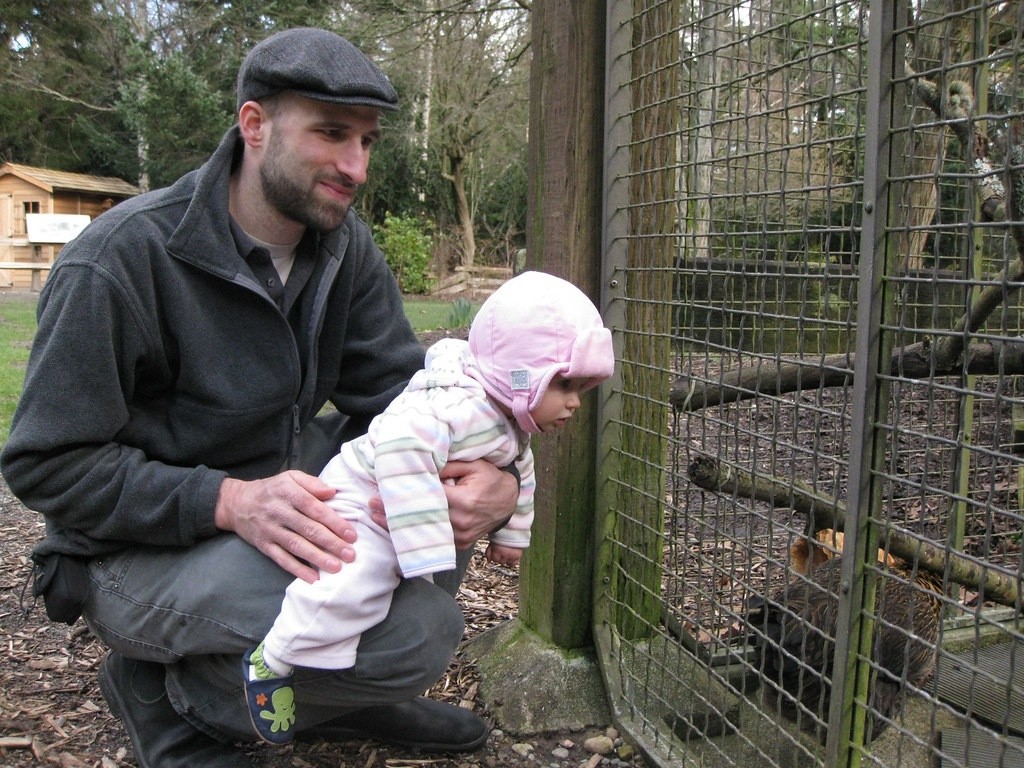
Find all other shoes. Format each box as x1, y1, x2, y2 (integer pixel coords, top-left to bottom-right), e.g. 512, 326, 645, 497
240, 645, 297, 747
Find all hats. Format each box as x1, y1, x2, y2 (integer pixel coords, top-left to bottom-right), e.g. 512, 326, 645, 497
236, 29, 400, 122
463, 269, 614, 434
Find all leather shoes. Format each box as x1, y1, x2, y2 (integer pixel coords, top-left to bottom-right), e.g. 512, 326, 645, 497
98, 649, 255, 768
294, 695, 489, 753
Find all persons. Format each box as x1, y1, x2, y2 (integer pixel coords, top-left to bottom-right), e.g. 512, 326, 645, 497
243, 270, 616, 746
0, 28, 521, 768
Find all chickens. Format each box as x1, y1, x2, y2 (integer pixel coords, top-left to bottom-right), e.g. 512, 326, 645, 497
747, 526, 943, 747
